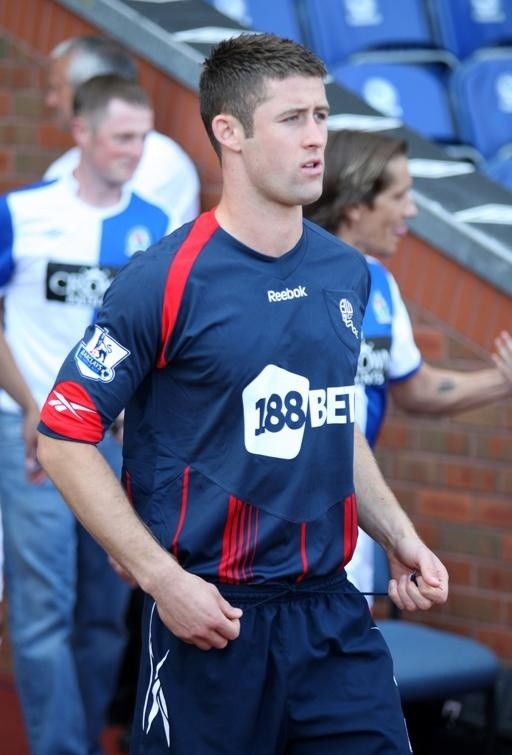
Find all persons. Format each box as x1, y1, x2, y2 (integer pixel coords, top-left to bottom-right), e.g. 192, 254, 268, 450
42, 35, 202, 236
301, 128, 512, 609
0, 71, 176, 753
34, 31, 451, 755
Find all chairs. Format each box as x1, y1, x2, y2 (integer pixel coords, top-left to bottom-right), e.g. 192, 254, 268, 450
336, 519, 501, 753
209, 0, 512, 193
